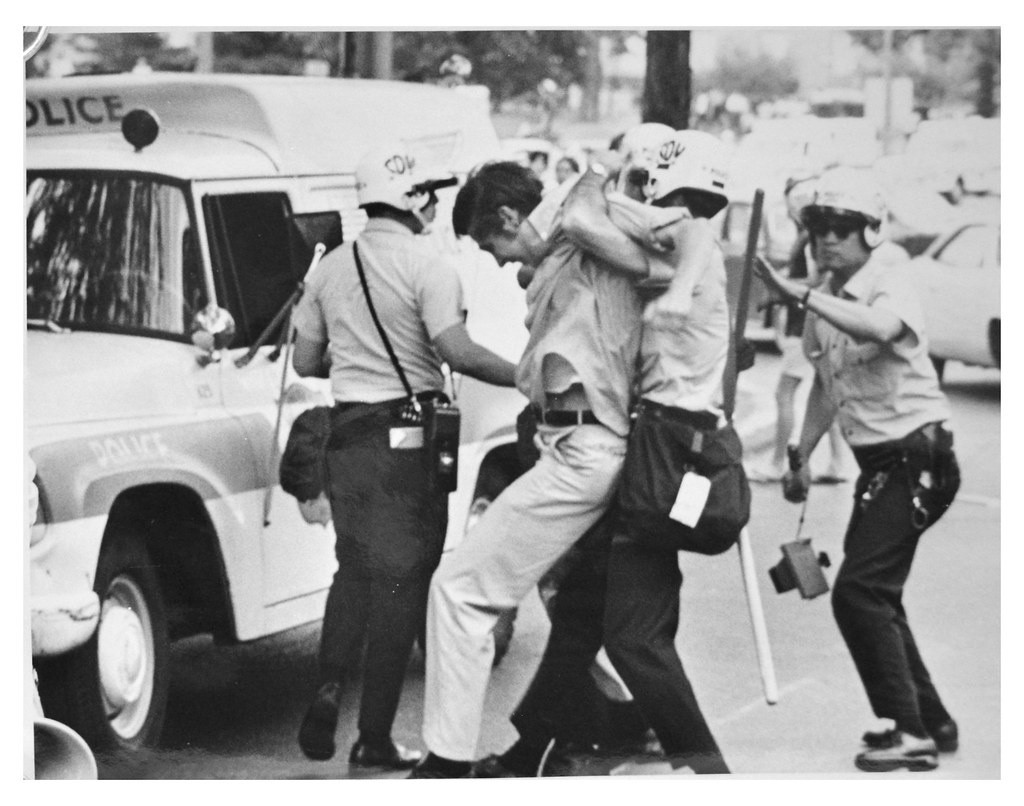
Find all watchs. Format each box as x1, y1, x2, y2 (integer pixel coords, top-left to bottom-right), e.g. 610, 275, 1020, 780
587, 163, 611, 179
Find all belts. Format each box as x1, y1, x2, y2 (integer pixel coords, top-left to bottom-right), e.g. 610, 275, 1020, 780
635, 397, 718, 429
536, 408, 600, 426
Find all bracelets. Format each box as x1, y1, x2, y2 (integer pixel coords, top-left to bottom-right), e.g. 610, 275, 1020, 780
793, 287, 811, 312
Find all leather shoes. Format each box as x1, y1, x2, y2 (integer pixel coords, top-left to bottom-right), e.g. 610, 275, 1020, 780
299, 681, 342, 762
861, 719, 960, 753
856, 734, 936, 774
350, 741, 422, 775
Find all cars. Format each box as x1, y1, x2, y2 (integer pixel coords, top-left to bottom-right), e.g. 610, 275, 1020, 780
493, 106, 998, 386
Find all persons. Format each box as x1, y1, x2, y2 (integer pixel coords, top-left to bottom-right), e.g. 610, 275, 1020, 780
400, 50, 811, 205
739, 167, 851, 487
471, 128, 733, 775
409, 158, 713, 776
746, 166, 962, 773
292, 141, 521, 779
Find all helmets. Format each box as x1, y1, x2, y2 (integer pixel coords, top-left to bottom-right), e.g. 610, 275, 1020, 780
643, 129, 731, 215
620, 124, 674, 182
355, 144, 456, 214
802, 171, 889, 246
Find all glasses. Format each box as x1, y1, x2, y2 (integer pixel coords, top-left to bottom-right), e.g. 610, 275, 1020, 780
810, 214, 862, 239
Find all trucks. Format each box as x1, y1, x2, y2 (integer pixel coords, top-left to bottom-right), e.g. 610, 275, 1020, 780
25, 67, 536, 767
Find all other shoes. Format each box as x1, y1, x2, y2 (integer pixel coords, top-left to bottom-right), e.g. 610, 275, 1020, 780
471, 755, 609, 776
